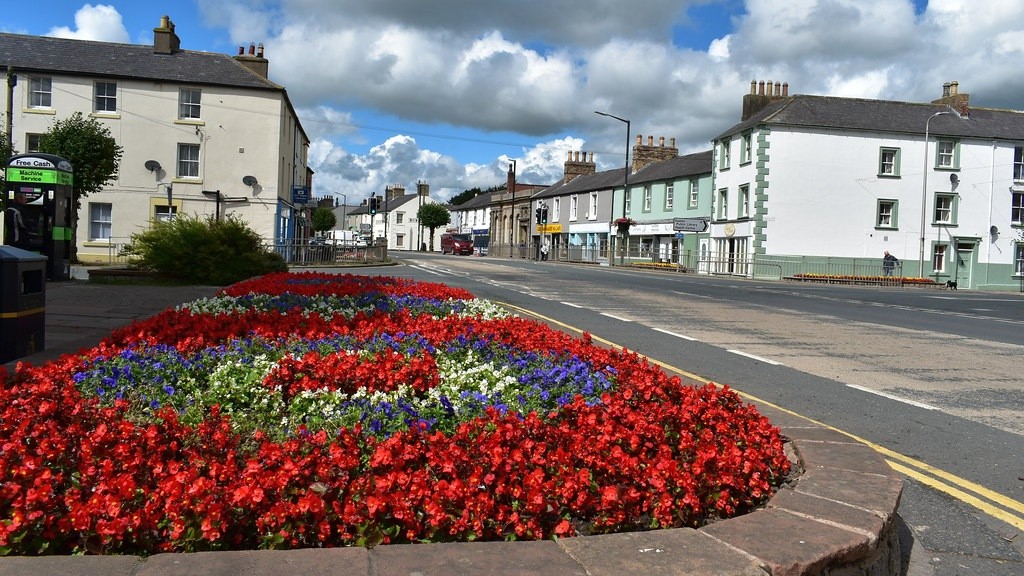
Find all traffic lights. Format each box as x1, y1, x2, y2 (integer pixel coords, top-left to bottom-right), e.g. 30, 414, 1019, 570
535, 208, 541, 223
541, 209, 547, 224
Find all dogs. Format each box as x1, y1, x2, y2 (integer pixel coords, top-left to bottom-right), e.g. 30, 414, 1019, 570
947, 280, 957, 290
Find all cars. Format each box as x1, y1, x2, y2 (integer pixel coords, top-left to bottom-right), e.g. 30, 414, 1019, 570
307, 236, 374, 254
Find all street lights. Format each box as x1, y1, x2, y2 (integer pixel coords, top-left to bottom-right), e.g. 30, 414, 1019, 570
334, 191, 346, 230
508, 159, 517, 258
369, 197, 377, 215
594, 110, 631, 266
919, 111, 950, 280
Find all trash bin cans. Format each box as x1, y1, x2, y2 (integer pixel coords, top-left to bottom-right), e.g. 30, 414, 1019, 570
1, 243, 48, 366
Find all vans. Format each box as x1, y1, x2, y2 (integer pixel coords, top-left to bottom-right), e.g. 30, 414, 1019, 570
441, 234, 473, 256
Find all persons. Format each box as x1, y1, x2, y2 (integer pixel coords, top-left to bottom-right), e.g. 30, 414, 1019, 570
543, 244, 549, 260
883, 251, 899, 277
540, 244, 546, 260
4, 193, 32, 250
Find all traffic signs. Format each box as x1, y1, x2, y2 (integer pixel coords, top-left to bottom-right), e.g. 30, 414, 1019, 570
673, 217, 709, 232
